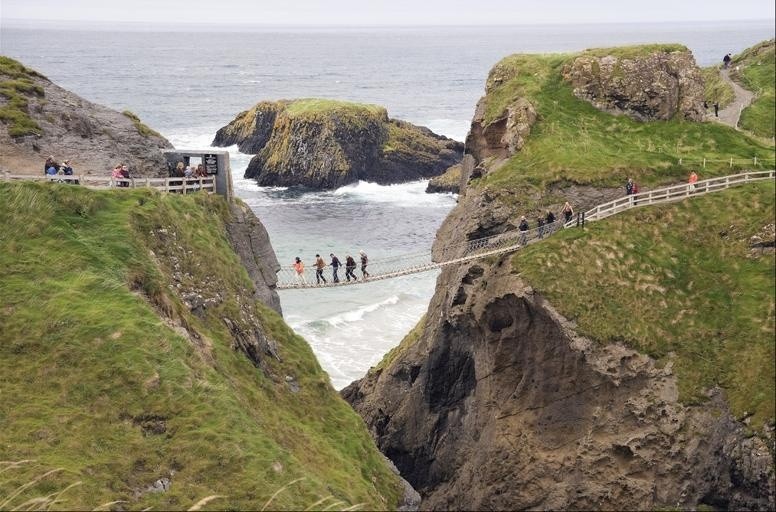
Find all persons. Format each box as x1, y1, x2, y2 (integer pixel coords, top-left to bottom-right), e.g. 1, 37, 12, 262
687, 169, 698, 197
344, 254, 357, 283
43, 155, 206, 193
560, 202, 574, 227
704, 101, 711, 115
536, 211, 545, 239
724, 53, 732, 70
713, 101, 719, 117
312, 254, 328, 285
329, 253, 343, 284
289, 256, 308, 286
359, 250, 370, 278
518, 215, 530, 245
625, 179, 639, 206
545, 209, 556, 235
